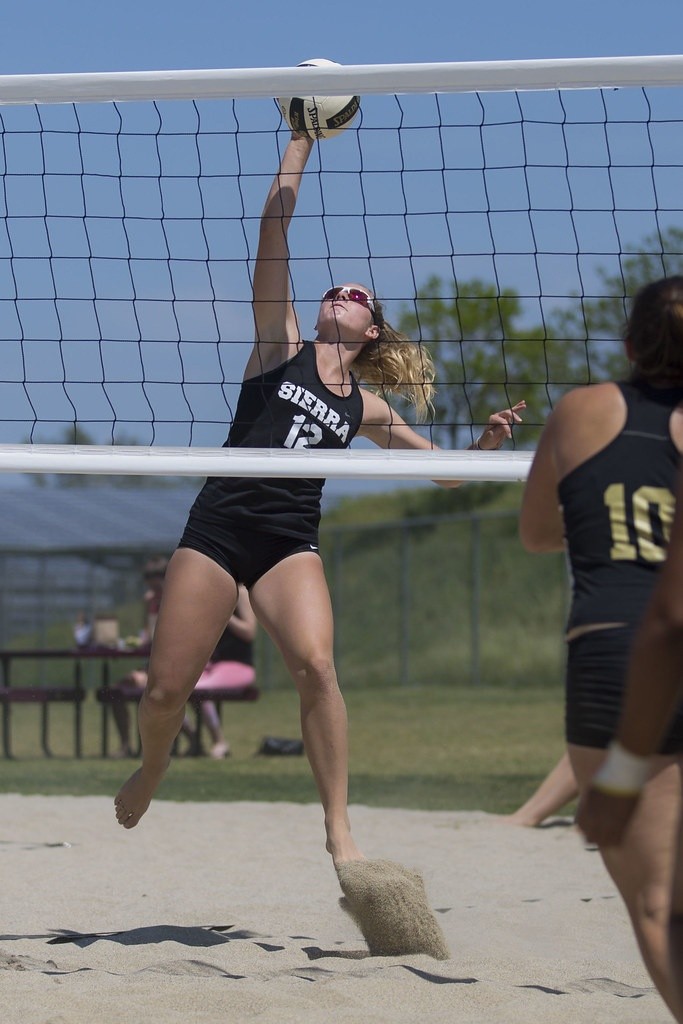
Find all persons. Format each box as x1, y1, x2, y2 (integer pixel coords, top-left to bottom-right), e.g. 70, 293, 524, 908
510, 275, 683, 1024
114, 132, 526, 862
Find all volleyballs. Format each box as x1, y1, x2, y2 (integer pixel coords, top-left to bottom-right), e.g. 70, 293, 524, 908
274, 55, 362, 142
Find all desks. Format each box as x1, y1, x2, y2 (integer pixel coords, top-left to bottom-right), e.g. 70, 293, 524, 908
0, 647, 151, 755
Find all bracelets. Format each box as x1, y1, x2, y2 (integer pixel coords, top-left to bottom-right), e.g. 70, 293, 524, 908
475, 436, 481, 450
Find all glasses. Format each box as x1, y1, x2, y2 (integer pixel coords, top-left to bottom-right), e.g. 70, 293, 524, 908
322, 286, 375, 325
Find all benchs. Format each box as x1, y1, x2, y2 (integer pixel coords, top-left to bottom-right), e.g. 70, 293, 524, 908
0, 686, 87, 760
97, 687, 261, 754
